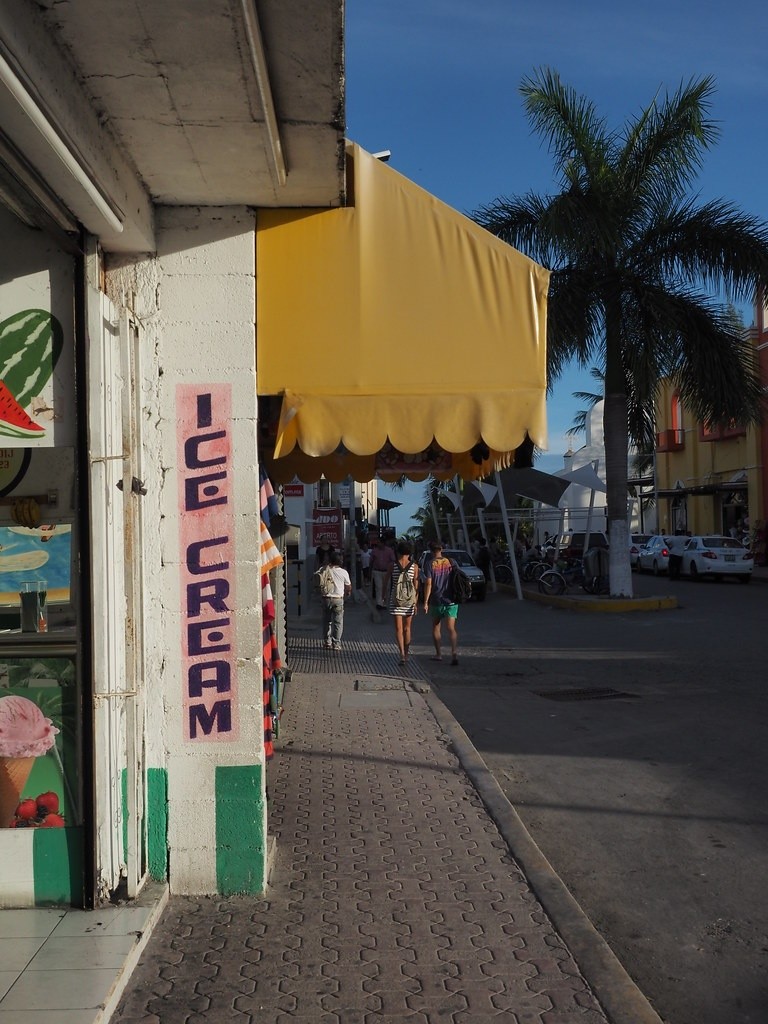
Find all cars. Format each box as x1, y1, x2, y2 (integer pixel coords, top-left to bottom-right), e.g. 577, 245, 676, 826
680, 536, 754, 584
416, 549, 486, 604
637, 535, 674, 576
628, 533, 655, 567
535, 531, 609, 578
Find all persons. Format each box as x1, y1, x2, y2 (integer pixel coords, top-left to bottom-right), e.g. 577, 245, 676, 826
736, 519, 745, 543
514, 535, 534, 557
320, 551, 352, 651
667, 529, 689, 581
361, 544, 373, 586
382, 542, 418, 664
661, 529, 665, 536
315, 535, 335, 569
441, 537, 500, 583
544, 532, 550, 541
422, 541, 459, 666
368, 537, 397, 611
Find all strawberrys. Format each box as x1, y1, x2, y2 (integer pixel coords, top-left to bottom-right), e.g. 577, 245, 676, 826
10, 790, 66, 828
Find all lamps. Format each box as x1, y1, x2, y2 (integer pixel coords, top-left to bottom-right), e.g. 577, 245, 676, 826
0, 55, 123, 234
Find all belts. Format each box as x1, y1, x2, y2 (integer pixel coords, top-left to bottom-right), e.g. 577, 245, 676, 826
373, 568, 388, 572
323, 597, 342, 599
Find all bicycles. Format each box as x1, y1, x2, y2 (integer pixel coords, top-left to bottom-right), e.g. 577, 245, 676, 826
494, 551, 606, 596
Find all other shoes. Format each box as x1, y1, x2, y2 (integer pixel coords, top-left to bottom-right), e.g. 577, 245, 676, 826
332, 643, 342, 650
322, 642, 333, 650
376, 604, 383, 610
399, 658, 405, 664
405, 649, 409, 662
452, 658, 458, 665
383, 604, 387, 609
430, 656, 443, 660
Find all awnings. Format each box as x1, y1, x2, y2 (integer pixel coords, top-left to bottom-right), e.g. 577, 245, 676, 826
256, 137, 552, 486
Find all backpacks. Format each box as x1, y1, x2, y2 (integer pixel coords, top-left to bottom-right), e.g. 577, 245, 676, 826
446, 556, 471, 603
394, 559, 417, 608
477, 546, 490, 566
312, 563, 334, 595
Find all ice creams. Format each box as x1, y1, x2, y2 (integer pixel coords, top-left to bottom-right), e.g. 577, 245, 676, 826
0, 695, 60, 828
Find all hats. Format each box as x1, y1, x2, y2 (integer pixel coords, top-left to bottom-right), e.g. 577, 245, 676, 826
536, 545, 542, 551
